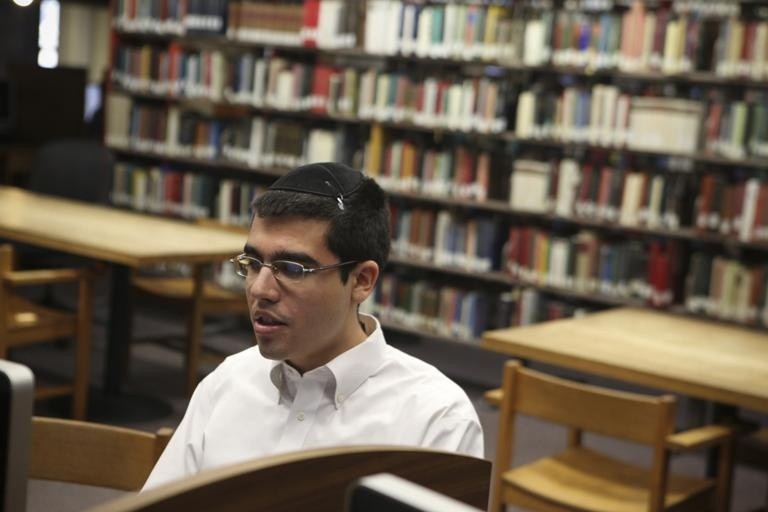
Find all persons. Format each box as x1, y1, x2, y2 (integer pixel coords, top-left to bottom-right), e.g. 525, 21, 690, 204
139, 163, 486, 495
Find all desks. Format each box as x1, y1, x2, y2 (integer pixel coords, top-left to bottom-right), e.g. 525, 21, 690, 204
478, 302, 766, 507
1, 182, 249, 416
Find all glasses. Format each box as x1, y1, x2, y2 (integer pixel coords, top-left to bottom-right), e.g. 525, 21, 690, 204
229, 251, 361, 285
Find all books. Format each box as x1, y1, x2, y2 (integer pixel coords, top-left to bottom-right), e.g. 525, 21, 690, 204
107, 1, 767, 345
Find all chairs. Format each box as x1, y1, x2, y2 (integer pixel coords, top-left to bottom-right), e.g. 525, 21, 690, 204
486, 358, 763, 512
1, 244, 92, 422
